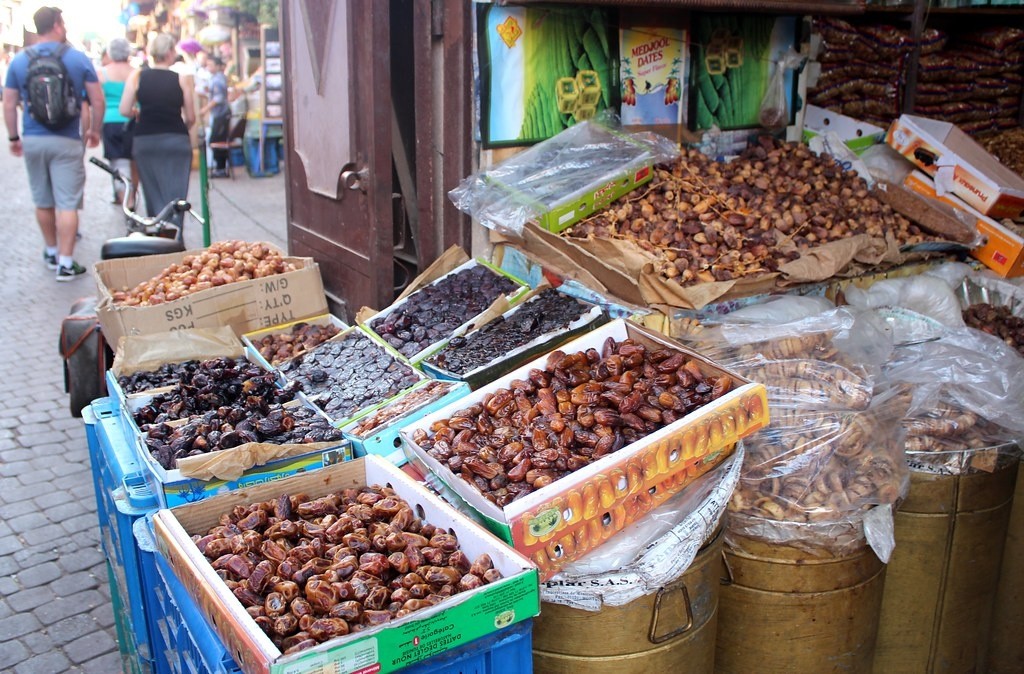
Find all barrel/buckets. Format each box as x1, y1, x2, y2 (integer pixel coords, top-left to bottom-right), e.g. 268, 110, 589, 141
532, 511, 726, 674
865, 450, 1024, 674
716, 502, 889, 674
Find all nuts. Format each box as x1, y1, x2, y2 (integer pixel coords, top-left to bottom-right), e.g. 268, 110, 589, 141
108, 136, 1024, 658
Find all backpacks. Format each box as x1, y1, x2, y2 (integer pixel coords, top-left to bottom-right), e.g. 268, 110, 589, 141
24, 42, 80, 131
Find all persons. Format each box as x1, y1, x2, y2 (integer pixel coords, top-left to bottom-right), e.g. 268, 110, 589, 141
92, 29, 226, 115
200, 57, 232, 175
3, 7, 106, 281
119, 35, 196, 240
97, 38, 142, 222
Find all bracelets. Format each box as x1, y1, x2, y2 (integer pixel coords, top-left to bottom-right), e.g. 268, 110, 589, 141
8, 136, 19, 141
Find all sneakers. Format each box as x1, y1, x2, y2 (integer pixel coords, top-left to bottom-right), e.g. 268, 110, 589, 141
56, 261, 87, 281
44, 250, 57, 270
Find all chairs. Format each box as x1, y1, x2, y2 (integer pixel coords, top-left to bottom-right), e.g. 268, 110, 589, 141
210, 113, 246, 181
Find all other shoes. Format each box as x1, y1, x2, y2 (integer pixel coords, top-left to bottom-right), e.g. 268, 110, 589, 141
208, 170, 227, 177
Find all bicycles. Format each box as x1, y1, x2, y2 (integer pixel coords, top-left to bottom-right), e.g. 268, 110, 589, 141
57, 157, 206, 420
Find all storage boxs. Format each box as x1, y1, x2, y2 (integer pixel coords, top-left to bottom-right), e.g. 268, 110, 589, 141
229, 149, 245, 167
80, 246, 771, 674
486, 118, 654, 234
901, 114, 1024, 198
796, 103, 885, 156
903, 174, 1024, 279
883, 119, 1024, 222
912, 170, 1024, 243
